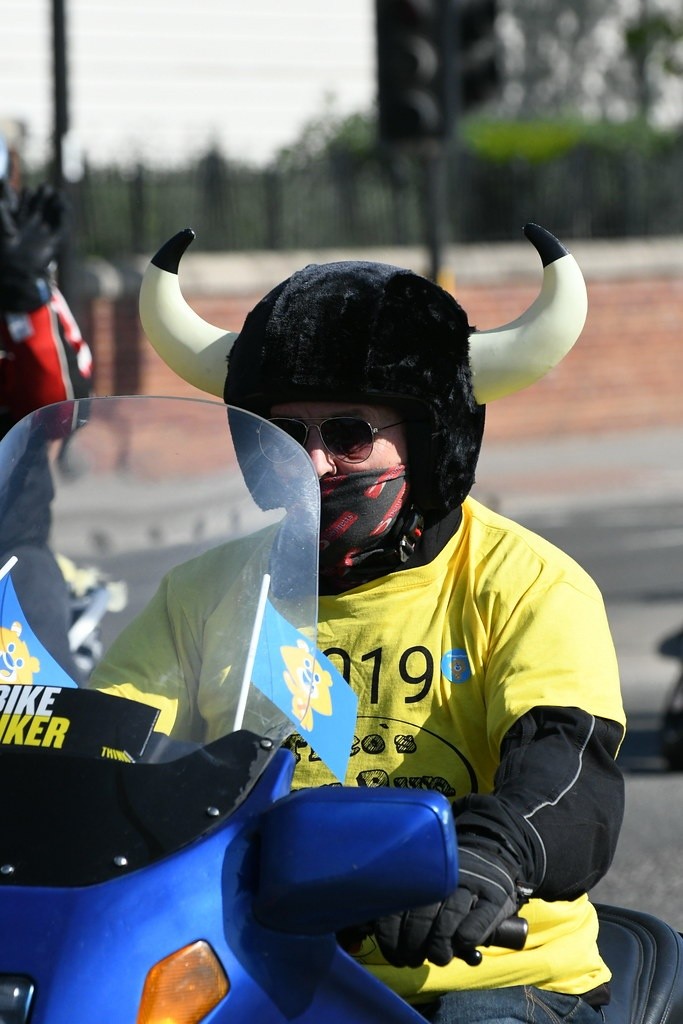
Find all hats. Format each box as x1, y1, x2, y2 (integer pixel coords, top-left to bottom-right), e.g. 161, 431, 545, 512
139, 221, 588, 533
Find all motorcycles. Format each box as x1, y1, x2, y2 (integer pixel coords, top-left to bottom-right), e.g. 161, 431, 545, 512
0, 394, 683, 1024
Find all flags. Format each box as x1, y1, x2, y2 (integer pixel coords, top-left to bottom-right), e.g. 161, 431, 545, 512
251, 600, 358, 783
0, 574, 78, 689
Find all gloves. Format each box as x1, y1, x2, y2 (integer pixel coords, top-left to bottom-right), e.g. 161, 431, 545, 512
337, 831, 537, 969
0, 179, 70, 312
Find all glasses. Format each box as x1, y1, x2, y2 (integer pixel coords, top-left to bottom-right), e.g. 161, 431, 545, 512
255, 414, 403, 465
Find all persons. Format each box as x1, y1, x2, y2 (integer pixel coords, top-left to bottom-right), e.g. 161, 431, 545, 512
0, 179, 94, 682
82, 222, 628, 1024
